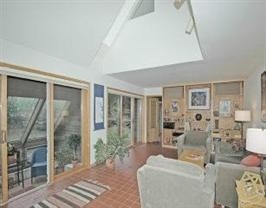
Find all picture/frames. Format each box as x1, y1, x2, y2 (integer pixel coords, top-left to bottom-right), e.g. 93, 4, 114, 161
188, 88, 210, 109
261, 71, 266, 123
219, 99, 232, 117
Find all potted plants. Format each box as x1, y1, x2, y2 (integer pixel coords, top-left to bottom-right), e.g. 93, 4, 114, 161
7, 142, 16, 164
66, 134, 81, 168
93, 128, 130, 168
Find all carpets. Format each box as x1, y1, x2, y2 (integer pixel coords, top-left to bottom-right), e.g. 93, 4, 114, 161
28, 179, 110, 208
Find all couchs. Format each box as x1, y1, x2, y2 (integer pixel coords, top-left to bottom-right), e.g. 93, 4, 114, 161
177, 130, 212, 163
137, 154, 216, 208
214, 150, 263, 208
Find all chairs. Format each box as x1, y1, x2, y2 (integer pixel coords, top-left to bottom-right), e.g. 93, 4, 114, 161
30, 147, 47, 183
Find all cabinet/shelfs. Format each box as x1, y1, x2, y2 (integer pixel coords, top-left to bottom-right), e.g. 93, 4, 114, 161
212, 79, 243, 148
235, 179, 266, 208
162, 84, 185, 148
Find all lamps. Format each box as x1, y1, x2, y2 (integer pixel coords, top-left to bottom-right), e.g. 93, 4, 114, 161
245, 128, 266, 196
234, 110, 251, 145
